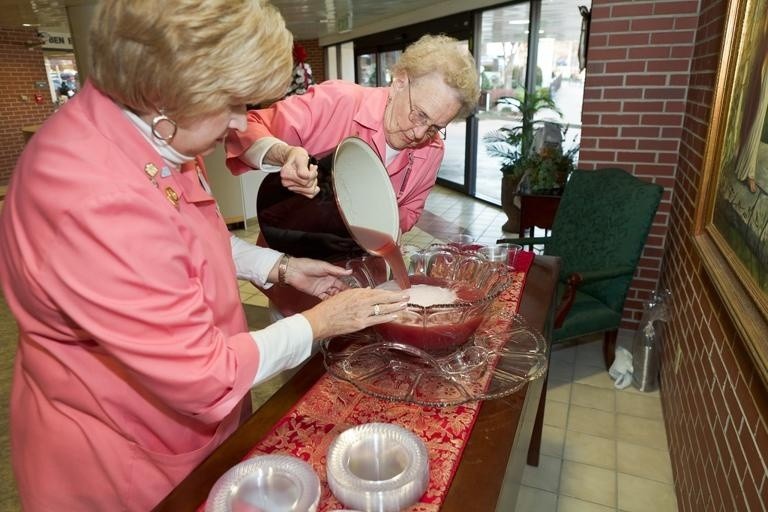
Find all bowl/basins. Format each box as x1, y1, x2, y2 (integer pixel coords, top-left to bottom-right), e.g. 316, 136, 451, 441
345, 242, 513, 355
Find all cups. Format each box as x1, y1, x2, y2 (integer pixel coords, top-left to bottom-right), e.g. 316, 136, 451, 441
446, 234, 523, 271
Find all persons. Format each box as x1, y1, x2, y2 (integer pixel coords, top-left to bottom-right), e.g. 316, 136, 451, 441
222, 36, 484, 383
0, 1, 409, 512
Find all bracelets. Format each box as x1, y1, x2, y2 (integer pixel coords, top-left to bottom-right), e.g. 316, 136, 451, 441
278, 253, 289, 288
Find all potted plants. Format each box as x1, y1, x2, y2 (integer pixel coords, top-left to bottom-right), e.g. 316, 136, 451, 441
480, 82, 584, 234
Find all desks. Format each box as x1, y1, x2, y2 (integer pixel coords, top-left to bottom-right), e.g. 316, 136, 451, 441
148, 249, 565, 511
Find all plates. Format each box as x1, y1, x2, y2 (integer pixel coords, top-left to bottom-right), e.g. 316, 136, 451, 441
205, 454, 321, 512
326, 422, 430, 511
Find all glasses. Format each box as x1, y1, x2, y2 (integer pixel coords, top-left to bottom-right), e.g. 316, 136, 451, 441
407, 73, 449, 146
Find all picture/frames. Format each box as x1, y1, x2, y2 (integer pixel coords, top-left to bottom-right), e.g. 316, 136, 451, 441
691, 1, 768, 396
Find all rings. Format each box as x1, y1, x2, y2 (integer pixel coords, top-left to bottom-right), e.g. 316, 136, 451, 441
374, 303, 380, 317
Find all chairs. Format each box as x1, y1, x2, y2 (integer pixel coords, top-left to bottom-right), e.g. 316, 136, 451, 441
496, 166, 664, 374
